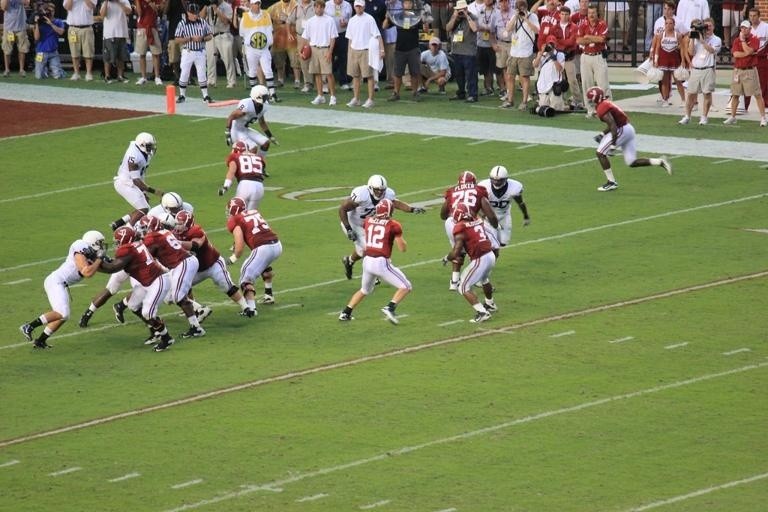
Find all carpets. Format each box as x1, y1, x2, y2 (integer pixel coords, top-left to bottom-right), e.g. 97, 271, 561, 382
612, 81, 767, 117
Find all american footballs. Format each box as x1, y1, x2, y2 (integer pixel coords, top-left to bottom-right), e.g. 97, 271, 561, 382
301, 45, 311, 61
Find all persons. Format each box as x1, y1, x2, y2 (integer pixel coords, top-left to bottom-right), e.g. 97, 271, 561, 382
0, 0, 767, 127
80, 193, 255, 352
439, 170, 504, 291
337, 198, 413, 326
337, 174, 426, 285
218, 85, 282, 316
475, 165, 530, 258
20, 231, 108, 349
442, 203, 497, 323
109, 132, 164, 234
585, 86, 671, 192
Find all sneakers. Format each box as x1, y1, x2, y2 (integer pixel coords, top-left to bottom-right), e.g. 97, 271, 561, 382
389, 88, 447, 103
660, 155, 672, 175
176, 92, 214, 106
19, 294, 275, 352
662, 99, 767, 126
277, 79, 353, 93
2, 71, 164, 88
307, 92, 377, 111
337, 303, 402, 325
448, 279, 497, 324
342, 254, 355, 281
263, 171, 270, 177
597, 180, 619, 191
448, 87, 530, 112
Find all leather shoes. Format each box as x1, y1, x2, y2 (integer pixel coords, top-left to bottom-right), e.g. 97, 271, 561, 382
74, 26, 90, 28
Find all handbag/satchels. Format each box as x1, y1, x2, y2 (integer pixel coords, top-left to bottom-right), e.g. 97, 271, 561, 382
560, 78, 569, 92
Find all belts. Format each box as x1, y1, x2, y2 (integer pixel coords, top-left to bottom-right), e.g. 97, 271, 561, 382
585, 52, 600, 56
266, 239, 277, 244
695, 66, 715, 70
213, 32, 223, 37
742, 67, 754, 70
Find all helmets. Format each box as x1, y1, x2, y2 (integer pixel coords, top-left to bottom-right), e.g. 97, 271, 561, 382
452, 165, 508, 221
83, 190, 246, 246
586, 87, 609, 114
367, 172, 396, 219
232, 141, 251, 156
135, 130, 157, 157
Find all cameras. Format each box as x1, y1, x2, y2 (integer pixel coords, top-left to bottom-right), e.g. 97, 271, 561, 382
458, 11, 464, 15
519, 11, 525, 16
545, 42, 554, 52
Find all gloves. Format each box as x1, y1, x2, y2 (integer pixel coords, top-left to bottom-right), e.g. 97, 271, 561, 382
594, 133, 604, 143
608, 145, 617, 156
496, 224, 504, 231
81, 246, 96, 258
523, 219, 530, 225
95, 247, 107, 258
226, 254, 238, 265
346, 225, 357, 240
271, 137, 278, 146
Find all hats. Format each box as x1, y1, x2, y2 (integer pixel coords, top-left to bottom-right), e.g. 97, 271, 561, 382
354, 0, 366, 7
546, 35, 557, 44
740, 19, 751, 28
187, 2, 200, 14
430, 37, 442, 45
453, 0, 468, 10
250, 0, 262, 4
